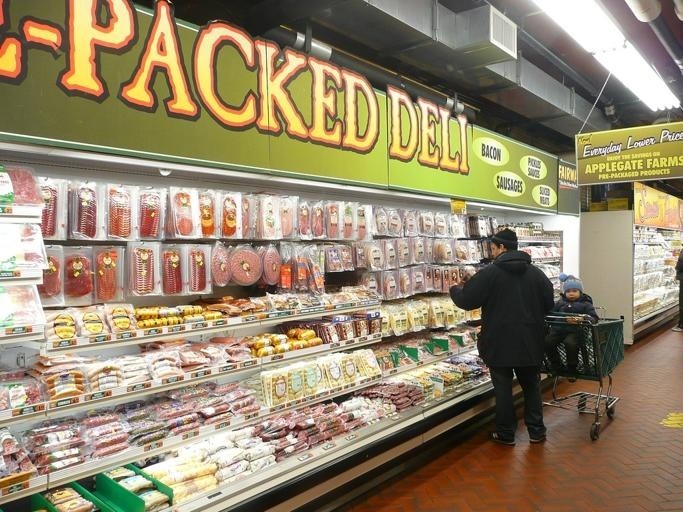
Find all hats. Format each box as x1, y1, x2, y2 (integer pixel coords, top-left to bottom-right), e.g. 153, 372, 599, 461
492, 228, 518, 245
559, 274, 583, 294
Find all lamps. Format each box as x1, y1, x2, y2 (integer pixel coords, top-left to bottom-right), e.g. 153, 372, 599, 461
532, 0, 681, 113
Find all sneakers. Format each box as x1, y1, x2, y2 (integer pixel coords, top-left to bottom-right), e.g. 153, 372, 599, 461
489, 430, 516, 446
529, 432, 547, 442
671, 324, 683, 332
567, 377, 576, 382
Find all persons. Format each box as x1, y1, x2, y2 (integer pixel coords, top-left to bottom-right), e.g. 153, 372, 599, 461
672, 244, 683, 332
544, 273, 599, 384
448, 228, 555, 447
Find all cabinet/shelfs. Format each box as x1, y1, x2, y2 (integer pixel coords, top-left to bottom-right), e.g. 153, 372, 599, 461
631, 224, 681, 337
1, 144, 567, 511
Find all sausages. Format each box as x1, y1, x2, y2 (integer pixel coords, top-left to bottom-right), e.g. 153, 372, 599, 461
1, 303, 427, 512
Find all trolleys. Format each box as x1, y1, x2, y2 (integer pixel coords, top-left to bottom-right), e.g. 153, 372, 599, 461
539, 307, 624, 441
